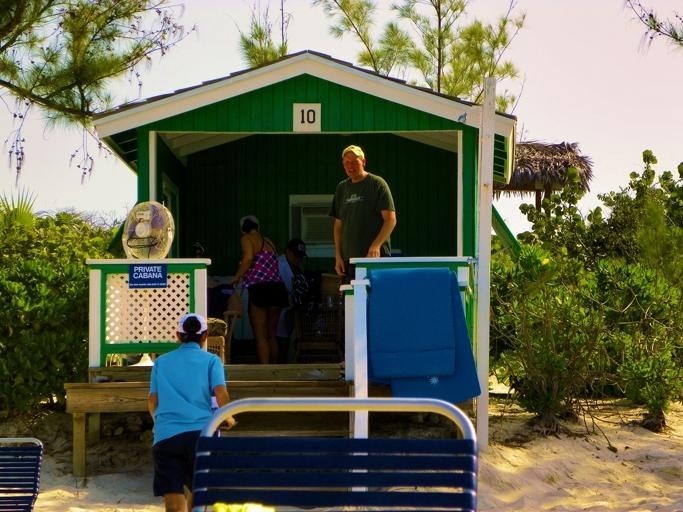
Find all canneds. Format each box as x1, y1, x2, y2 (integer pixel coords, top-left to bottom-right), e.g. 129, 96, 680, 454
326, 296, 334, 307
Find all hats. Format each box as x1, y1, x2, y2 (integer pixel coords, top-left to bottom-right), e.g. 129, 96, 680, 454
343, 145, 365, 160
240, 215, 260, 232
177, 313, 208, 334
288, 238, 307, 257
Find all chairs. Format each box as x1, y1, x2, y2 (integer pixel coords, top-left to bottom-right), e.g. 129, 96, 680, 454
208, 283, 242, 363
291, 273, 344, 363
206, 317, 228, 360
0, 437, 44, 512
188, 394, 482, 512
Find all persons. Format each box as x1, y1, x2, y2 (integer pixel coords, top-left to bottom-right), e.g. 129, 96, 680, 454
276, 237, 308, 362
146, 312, 236, 511
228, 213, 288, 363
326, 143, 397, 286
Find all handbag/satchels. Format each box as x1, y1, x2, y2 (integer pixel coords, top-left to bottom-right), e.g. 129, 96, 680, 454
227, 293, 242, 320
